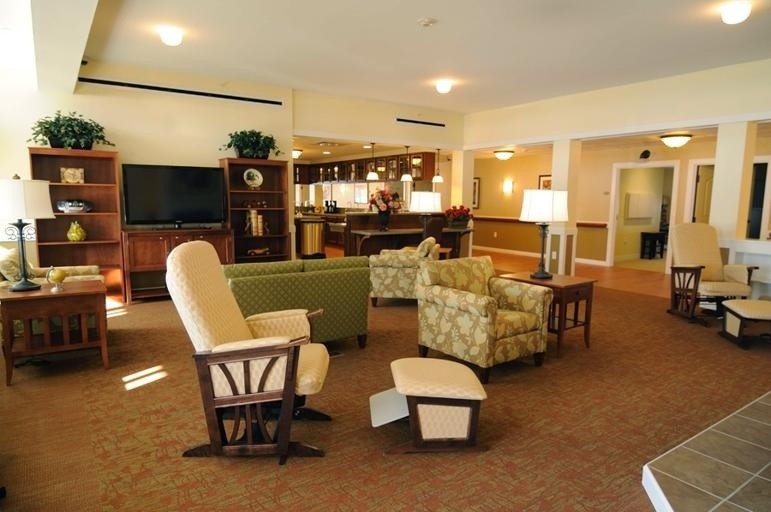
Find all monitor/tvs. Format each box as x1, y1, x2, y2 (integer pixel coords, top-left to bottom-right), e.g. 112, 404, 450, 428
121, 163, 226, 230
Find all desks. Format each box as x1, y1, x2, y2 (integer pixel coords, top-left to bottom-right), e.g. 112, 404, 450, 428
400, 241, 455, 261
499, 264, 599, 360
0, 274, 118, 387
636, 227, 666, 262
348, 225, 477, 256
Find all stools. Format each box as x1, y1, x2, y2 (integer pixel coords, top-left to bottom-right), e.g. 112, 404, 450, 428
361, 354, 494, 455
716, 292, 771, 351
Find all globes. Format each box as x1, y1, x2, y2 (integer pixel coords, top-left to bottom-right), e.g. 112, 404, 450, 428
46, 266, 66, 293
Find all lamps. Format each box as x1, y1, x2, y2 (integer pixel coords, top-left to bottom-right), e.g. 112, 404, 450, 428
514, 186, 580, 283
492, 148, 517, 164
659, 131, 694, 150
3, 169, 61, 295
408, 190, 445, 240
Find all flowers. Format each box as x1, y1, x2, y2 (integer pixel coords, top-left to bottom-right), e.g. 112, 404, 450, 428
441, 203, 476, 224
369, 189, 403, 213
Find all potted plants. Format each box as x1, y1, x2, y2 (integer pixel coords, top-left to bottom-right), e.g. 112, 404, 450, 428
26, 105, 119, 154
217, 127, 279, 161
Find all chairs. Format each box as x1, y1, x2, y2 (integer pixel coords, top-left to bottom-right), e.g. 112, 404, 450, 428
413, 251, 556, 383
156, 239, 339, 468
364, 235, 443, 308
664, 220, 761, 332
2, 242, 111, 341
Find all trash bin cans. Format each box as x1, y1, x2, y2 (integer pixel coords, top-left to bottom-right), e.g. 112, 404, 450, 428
299, 219, 328, 259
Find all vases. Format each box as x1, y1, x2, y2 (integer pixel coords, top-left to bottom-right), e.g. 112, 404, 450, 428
447, 218, 469, 230
378, 209, 392, 231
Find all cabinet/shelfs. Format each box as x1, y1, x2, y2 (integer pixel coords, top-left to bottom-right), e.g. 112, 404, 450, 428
293, 144, 445, 185
119, 224, 240, 310
214, 154, 296, 264
23, 142, 133, 306
303, 213, 348, 247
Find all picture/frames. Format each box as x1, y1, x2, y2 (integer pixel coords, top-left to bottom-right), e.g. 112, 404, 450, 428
537, 173, 553, 191
472, 175, 481, 212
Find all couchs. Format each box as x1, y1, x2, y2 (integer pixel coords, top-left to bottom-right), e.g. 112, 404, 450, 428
214, 249, 374, 357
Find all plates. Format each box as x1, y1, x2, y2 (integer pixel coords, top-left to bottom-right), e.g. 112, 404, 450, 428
242, 167, 264, 186
